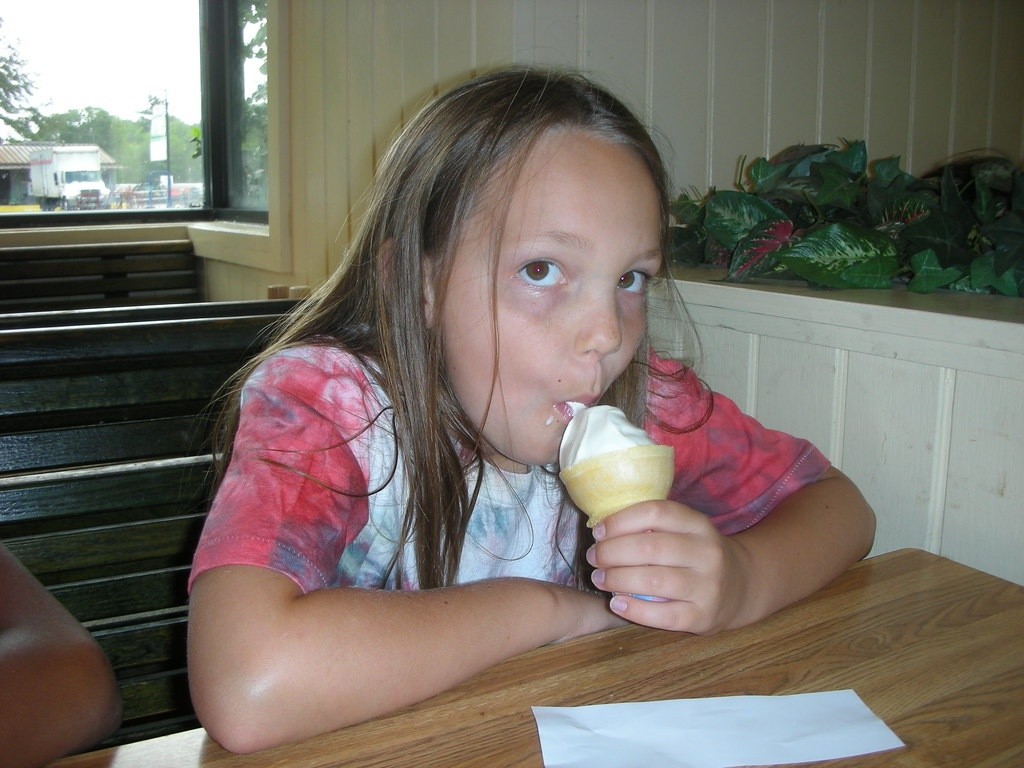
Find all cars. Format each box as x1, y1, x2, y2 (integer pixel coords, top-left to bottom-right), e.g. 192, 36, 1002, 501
58, 180, 204, 210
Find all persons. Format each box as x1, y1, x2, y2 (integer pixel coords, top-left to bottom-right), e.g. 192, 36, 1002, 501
0, 543, 121, 768
189, 63, 875, 753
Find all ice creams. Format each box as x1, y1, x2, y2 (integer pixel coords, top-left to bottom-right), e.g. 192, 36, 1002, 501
556, 402, 675, 525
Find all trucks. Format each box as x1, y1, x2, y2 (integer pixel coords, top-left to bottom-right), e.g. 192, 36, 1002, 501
28, 145, 118, 212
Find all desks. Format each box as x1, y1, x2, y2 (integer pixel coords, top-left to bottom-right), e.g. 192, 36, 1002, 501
32, 546, 1024, 767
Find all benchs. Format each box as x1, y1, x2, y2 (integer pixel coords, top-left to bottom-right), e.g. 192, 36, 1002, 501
0, 238, 320, 757
